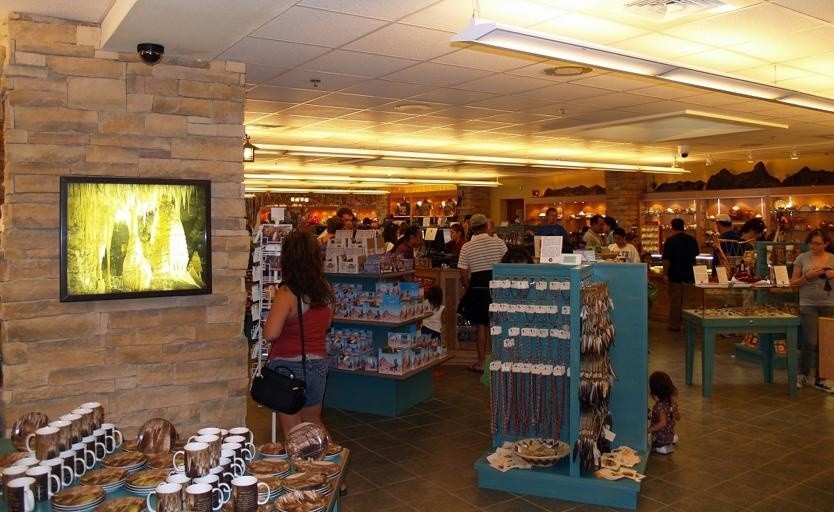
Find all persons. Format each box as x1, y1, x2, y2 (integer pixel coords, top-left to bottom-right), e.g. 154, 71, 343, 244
535, 208, 573, 252
730, 218, 765, 255
262, 230, 348, 496
647, 370, 681, 456
444, 224, 469, 258
569, 214, 641, 263
791, 228, 834, 392
457, 214, 509, 373
710, 214, 737, 338
422, 286, 448, 376
512, 215, 519, 223
641, 252, 660, 321
661, 218, 699, 330
310, 207, 423, 270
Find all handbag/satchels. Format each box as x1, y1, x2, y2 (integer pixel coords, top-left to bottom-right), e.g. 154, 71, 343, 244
251, 367, 306, 415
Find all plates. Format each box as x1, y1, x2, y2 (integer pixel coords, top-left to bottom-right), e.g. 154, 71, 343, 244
270, 422, 343, 512
570, 212, 606, 219
123, 418, 186, 512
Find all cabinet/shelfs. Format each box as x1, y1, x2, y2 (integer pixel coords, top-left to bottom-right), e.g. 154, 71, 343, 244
640, 186, 834, 398
248, 183, 492, 419
474, 237, 653, 512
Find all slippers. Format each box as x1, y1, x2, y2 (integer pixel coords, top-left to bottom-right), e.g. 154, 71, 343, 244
340, 488, 347, 496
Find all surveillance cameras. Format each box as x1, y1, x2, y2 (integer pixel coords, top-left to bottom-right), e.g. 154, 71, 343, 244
678, 145, 690, 158
137, 44, 164, 68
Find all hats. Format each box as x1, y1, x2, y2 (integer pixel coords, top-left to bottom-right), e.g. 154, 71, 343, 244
714, 213, 731, 222
470, 214, 487, 227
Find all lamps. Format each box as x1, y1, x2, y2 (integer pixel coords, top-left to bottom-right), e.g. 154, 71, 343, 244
244, 134, 258, 162
671, 144, 801, 170
451, 1, 834, 116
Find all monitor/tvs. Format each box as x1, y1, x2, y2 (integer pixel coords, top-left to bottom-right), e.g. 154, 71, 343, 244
442, 228, 454, 244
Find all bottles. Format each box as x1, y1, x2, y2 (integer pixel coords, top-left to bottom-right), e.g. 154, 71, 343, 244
739, 259, 745, 272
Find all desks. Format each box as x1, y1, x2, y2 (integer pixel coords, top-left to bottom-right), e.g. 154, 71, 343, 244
0, 413, 348, 511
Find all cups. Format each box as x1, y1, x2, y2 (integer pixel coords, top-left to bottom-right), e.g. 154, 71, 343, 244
186, 427, 270, 512
0, 402, 122, 512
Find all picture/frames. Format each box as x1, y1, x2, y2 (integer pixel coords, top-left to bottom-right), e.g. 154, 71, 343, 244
60, 175, 212, 304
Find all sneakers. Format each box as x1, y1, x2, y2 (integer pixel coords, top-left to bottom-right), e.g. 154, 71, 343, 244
797, 374, 806, 388
814, 380, 834, 393
656, 444, 674, 454
468, 363, 486, 371
672, 434, 678, 442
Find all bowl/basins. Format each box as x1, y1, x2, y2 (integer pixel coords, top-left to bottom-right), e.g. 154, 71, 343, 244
599, 253, 618, 259
512, 438, 570, 467
